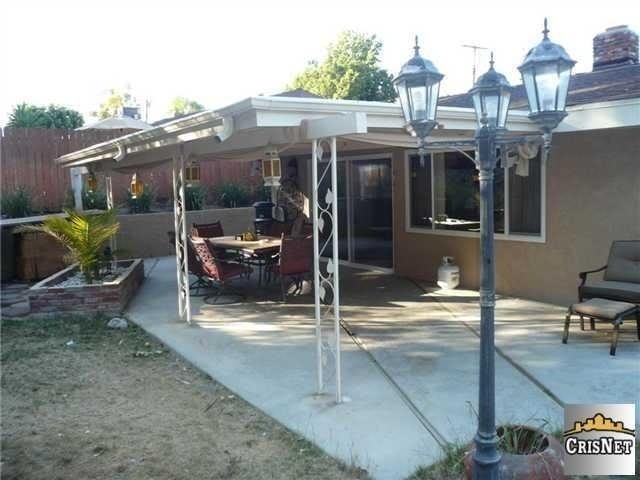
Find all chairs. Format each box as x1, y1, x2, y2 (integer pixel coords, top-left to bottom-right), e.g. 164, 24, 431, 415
168, 219, 313, 306
578, 240, 640, 330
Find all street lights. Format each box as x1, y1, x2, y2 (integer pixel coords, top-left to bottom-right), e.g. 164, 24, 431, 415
392, 18, 577, 479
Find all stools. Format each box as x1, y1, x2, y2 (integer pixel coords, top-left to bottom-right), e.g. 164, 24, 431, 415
562, 298, 640, 356
